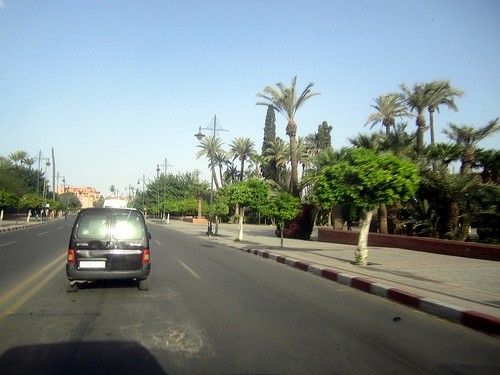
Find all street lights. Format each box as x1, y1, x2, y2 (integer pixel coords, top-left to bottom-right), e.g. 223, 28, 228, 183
56, 171, 65, 216
156, 158, 173, 221
194, 114, 230, 238
137, 173, 149, 215
34, 149, 51, 222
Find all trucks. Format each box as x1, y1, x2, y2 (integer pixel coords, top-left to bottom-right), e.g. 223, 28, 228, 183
102, 198, 127, 208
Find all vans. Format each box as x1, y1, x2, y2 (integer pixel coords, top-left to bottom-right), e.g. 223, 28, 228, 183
65, 207, 152, 290
